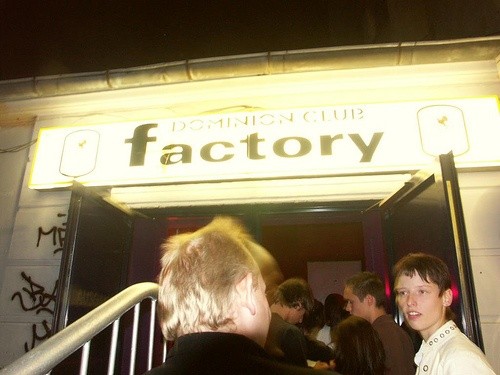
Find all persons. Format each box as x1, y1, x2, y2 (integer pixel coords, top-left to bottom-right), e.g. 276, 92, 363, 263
143, 214, 341, 375
333, 316, 390, 375
343, 272, 416, 375
393, 252, 497, 375
262, 276, 352, 374
